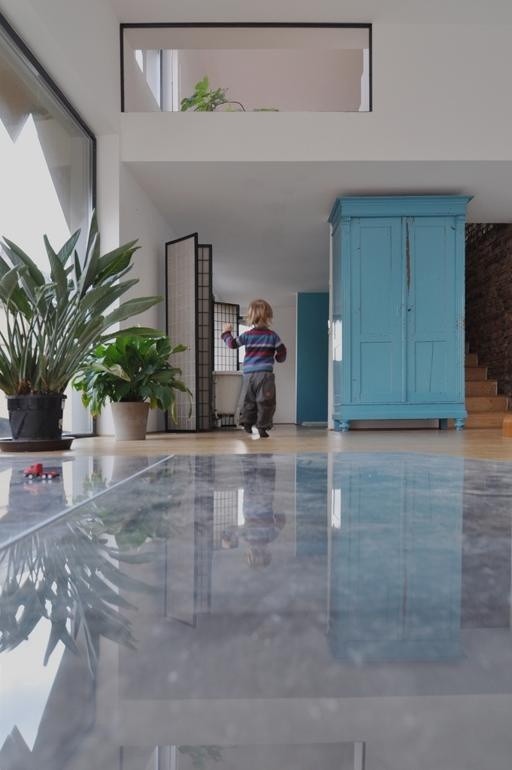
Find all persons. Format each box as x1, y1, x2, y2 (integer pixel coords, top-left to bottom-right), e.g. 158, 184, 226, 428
221, 299, 288, 437
220, 452, 286, 566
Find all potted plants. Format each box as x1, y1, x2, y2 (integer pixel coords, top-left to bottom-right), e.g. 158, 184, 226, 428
0, 455, 184, 675
0, 228, 161, 450
74, 327, 193, 441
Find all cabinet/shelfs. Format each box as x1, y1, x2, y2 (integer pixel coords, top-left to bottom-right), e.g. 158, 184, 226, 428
329, 192, 475, 432
